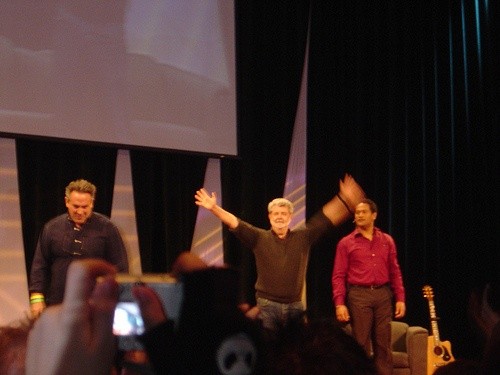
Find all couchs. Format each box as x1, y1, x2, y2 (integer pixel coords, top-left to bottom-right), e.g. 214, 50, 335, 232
346, 322, 428, 375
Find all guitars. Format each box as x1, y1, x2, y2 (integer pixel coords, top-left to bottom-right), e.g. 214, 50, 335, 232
423, 286, 455, 375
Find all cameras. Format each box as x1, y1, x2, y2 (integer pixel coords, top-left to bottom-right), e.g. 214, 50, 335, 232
111, 298, 146, 337
